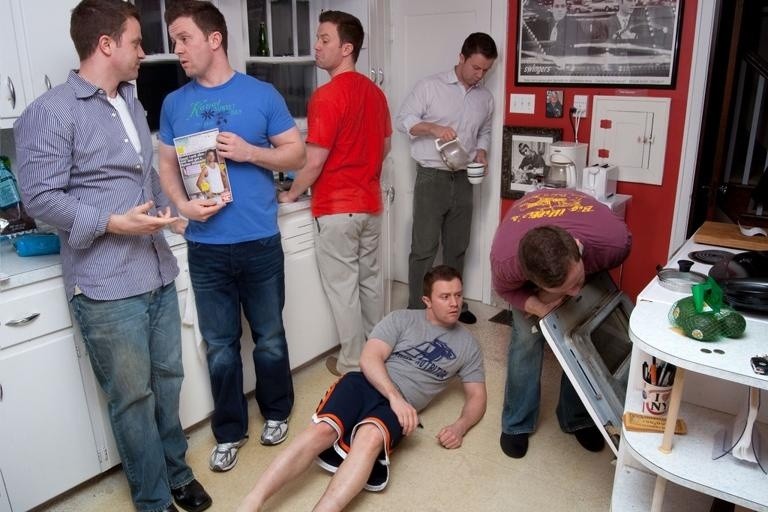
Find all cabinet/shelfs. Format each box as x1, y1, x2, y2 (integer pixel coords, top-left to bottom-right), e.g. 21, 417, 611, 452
0, 2, 139, 128
612, 301, 768, 511
83, 248, 260, 470
0, 284, 106, 512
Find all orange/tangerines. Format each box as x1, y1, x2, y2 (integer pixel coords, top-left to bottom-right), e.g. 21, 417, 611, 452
673, 296, 746, 341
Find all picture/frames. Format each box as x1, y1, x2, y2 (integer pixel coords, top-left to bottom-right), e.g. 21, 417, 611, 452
499, 124, 563, 201
513, 0, 686, 90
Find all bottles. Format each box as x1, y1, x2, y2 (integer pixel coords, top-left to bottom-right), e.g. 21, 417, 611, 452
256, 22, 269, 57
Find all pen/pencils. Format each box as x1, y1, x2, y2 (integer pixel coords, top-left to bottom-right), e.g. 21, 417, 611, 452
642, 356, 677, 387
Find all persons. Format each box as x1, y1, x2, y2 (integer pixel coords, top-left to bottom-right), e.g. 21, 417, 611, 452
278, 9, 394, 376
195, 147, 229, 200
538, 0, 590, 55
546, 91, 562, 118
393, 31, 499, 329
233, 264, 489, 512
12, 1, 213, 512
156, 1, 307, 473
600, 0, 651, 56
516, 142, 548, 185
487, 186, 635, 460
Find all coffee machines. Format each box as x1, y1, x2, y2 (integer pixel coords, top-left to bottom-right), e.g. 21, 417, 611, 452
542, 139, 588, 195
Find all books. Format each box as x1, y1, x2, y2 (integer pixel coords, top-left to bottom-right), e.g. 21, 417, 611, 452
172, 126, 234, 206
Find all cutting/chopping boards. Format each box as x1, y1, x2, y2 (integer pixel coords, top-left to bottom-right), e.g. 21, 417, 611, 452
693, 218, 767, 250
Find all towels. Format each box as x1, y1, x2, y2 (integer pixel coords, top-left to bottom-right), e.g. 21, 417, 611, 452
181, 270, 208, 364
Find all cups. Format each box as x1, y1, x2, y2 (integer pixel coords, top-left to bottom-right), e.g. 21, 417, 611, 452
642, 373, 679, 424
435, 135, 472, 174
468, 162, 484, 184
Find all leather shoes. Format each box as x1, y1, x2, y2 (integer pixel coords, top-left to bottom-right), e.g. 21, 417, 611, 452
159, 477, 213, 511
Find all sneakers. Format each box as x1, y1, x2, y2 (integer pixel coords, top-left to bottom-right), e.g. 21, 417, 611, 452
325, 355, 343, 377
500, 431, 529, 459
458, 309, 478, 324
208, 429, 249, 473
573, 425, 605, 453
259, 413, 291, 446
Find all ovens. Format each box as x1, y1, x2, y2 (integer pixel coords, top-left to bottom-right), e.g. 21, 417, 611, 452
537, 268, 638, 456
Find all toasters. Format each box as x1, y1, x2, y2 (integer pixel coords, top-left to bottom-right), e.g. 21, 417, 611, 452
583, 162, 619, 202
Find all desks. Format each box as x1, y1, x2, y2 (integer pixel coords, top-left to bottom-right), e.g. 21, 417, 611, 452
522, 184, 634, 297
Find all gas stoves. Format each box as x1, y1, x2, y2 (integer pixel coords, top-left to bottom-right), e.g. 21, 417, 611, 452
676, 240, 748, 274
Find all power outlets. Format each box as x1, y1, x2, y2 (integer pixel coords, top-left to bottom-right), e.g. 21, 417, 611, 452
573, 94, 589, 119
